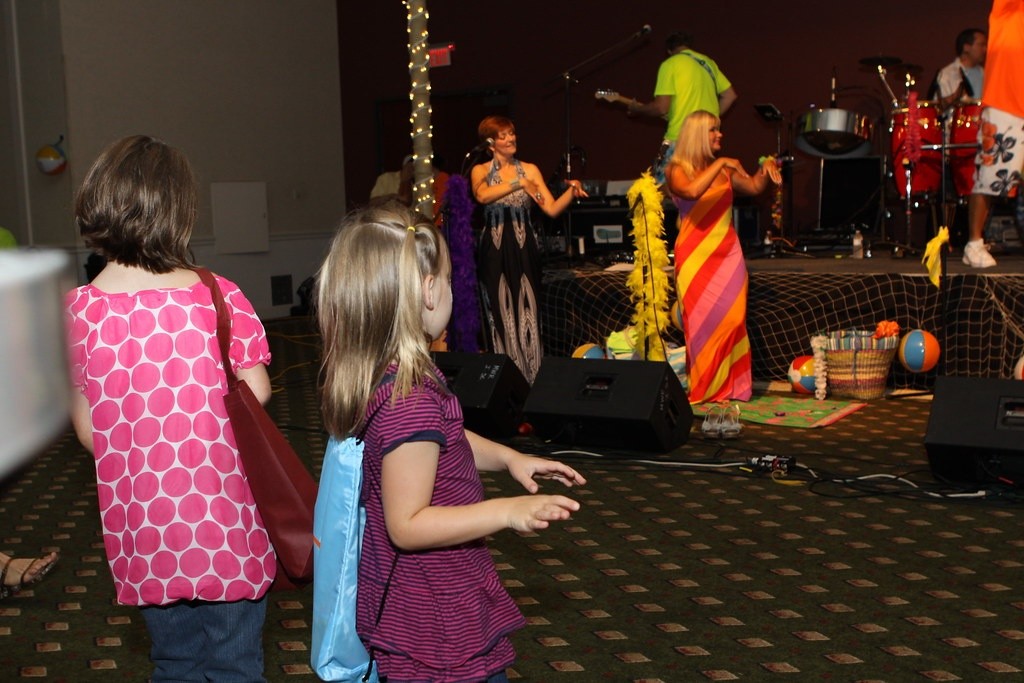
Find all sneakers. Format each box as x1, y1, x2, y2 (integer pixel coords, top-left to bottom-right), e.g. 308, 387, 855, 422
962, 238, 996, 269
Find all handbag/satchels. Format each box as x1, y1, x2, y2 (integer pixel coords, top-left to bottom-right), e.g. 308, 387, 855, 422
193, 268, 317, 579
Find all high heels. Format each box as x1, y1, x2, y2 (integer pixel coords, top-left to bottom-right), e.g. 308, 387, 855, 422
721, 405, 741, 437
702, 406, 721, 438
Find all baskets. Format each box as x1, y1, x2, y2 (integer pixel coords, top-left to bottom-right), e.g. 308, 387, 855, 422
822, 332, 897, 399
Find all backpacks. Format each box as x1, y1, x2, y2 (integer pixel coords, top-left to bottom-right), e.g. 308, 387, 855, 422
310, 371, 448, 683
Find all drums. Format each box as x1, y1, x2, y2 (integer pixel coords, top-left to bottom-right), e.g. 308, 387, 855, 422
950, 98, 984, 198
791, 108, 876, 159
891, 99, 946, 200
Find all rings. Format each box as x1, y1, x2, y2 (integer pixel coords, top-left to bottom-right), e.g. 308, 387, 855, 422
537, 194, 541, 199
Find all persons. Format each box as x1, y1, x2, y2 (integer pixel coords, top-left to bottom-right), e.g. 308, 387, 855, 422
933, 27, 985, 113
626, 33, 736, 181
962, 0, 1024, 268
65, 135, 271, 683
665, 109, 783, 398
315, 201, 586, 682
0, 552, 57, 598
471, 116, 588, 382
367, 160, 416, 208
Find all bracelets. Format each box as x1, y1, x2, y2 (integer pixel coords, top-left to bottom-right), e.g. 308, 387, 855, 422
511, 180, 519, 191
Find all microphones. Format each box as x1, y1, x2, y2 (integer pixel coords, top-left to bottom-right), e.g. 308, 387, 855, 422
465, 138, 495, 157
831, 71, 838, 108
959, 66, 974, 97
926, 70, 941, 101
636, 25, 652, 38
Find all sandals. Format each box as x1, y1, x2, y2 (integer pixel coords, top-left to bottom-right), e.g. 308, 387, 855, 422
0, 555, 60, 602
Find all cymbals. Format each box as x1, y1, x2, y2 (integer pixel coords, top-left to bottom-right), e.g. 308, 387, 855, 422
893, 63, 924, 73
859, 57, 903, 65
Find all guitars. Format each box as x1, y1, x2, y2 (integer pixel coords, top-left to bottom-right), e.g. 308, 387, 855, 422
593, 86, 669, 120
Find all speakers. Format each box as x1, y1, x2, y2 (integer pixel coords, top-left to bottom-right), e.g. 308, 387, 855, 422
521, 355, 694, 457
817, 155, 887, 234
924, 376, 1024, 497
569, 210, 637, 261
427, 349, 531, 445
